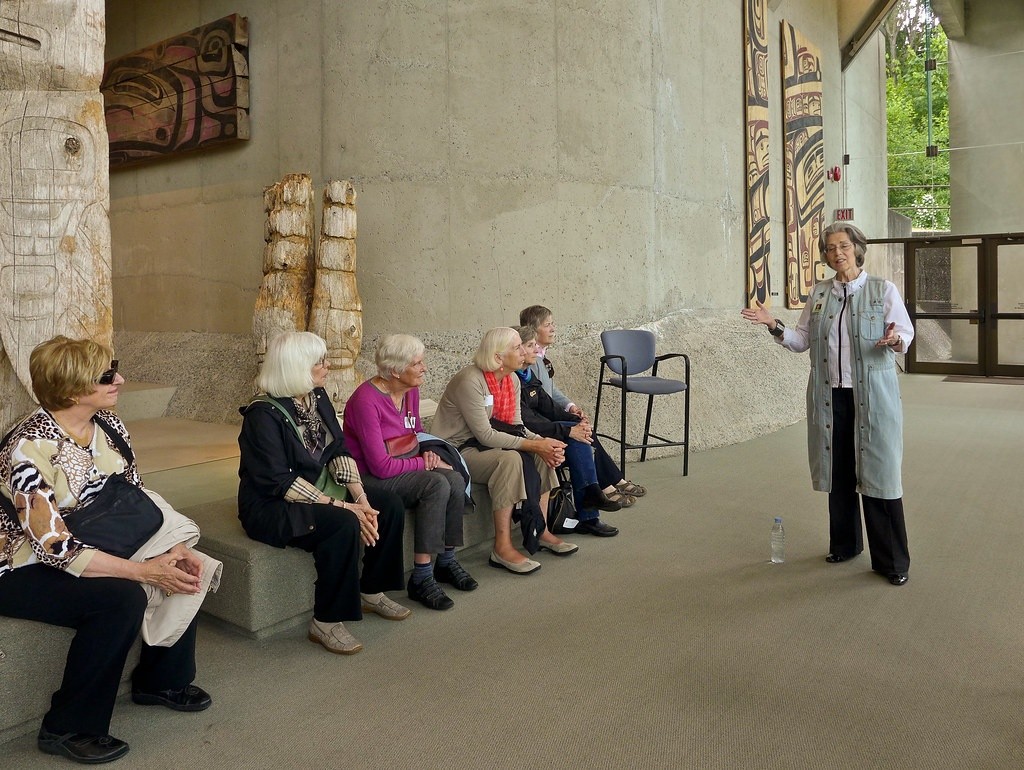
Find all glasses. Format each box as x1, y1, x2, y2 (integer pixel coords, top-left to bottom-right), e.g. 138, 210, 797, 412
543, 358, 554, 378
94, 360, 118, 384
314, 353, 327, 369
823, 241, 857, 254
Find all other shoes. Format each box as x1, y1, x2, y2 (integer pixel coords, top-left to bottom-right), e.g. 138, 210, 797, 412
536, 538, 579, 556
489, 548, 541, 575
308, 616, 362, 654
360, 594, 411, 620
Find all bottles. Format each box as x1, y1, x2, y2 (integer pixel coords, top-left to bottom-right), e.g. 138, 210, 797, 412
771, 518, 785, 563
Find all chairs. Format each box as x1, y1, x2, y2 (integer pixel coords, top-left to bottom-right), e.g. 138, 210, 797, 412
593, 330, 691, 480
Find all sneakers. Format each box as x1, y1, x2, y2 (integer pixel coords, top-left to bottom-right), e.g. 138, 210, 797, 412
407, 573, 454, 610
433, 553, 478, 590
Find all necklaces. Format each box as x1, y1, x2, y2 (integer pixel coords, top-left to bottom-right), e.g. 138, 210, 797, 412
382, 379, 403, 412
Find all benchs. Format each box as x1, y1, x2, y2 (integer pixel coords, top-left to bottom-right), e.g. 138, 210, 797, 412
195, 483, 521, 642
0, 616, 140, 746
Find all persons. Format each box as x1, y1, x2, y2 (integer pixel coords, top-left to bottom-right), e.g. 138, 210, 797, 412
520, 305, 648, 507
508, 326, 621, 537
342, 333, 478, 611
0, 335, 214, 764
741, 222, 915, 586
430, 327, 579, 575
237, 330, 411, 656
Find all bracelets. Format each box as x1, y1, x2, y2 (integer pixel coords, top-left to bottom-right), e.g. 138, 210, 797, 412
355, 493, 367, 503
888, 335, 902, 346
340, 500, 346, 508
329, 497, 335, 505
768, 319, 786, 337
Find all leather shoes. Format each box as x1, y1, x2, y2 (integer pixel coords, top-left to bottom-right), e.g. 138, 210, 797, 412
132, 684, 212, 711
889, 574, 907, 585
582, 493, 621, 512
826, 553, 843, 562
38, 726, 129, 763
574, 519, 618, 537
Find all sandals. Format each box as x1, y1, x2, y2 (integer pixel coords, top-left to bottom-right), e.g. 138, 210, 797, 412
614, 480, 647, 496
604, 489, 637, 507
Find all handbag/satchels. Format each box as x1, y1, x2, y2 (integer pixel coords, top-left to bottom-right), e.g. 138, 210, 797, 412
489, 417, 527, 438
384, 432, 420, 461
60, 472, 164, 560
547, 467, 580, 534
312, 463, 347, 502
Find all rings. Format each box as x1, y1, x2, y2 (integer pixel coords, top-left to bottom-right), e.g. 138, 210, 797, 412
166, 591, 173, 597
